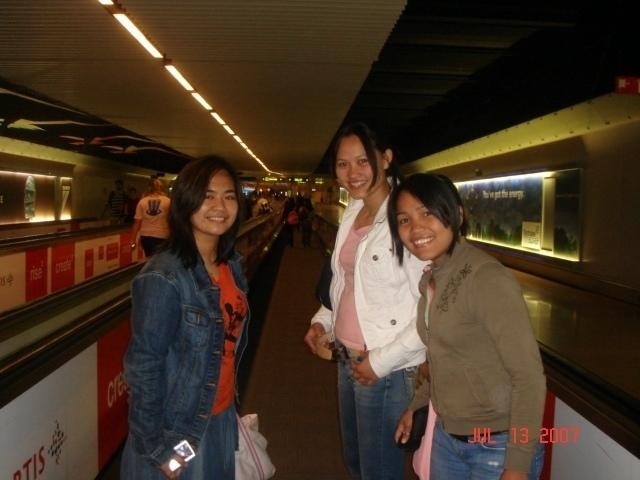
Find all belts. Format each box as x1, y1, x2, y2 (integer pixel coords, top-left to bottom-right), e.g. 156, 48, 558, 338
315, 329, 364, 360
447, 431, 508, 443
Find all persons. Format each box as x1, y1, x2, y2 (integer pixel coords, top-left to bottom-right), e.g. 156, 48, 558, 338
296, 198, 315, 248
118, 150, 256, 480
303, 118, 440, 479
244, 187, 285, 218
384, 171, 546, 479
124, 187, 140, 219
126, 177, 173, 257
279, 197, 298, 247
103, 180, 130, 222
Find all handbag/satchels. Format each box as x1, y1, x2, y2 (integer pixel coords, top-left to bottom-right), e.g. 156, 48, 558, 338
235, 413, 276, 480
398, 398, 437, 480
315, 257, 332, 311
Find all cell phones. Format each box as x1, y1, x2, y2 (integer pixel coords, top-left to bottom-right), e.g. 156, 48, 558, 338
168, 439, 195, 471
